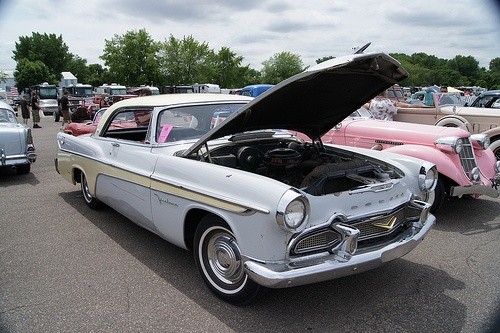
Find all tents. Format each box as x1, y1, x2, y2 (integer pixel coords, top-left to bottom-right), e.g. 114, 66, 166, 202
445, 86, 464, 97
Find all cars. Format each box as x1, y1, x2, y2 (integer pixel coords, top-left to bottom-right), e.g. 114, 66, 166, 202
54, 41, 500, 308
0, 102, 38, 175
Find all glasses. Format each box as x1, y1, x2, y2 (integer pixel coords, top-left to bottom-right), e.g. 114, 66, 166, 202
441, 91, 447, 92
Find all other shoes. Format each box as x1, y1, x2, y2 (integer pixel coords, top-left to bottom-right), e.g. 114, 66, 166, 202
33, 125, 42, 128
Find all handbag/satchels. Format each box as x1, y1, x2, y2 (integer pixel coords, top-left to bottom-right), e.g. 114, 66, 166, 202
39, 109, 45, 118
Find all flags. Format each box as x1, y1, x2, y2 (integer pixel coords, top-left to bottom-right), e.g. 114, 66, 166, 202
6, 86, 19, 96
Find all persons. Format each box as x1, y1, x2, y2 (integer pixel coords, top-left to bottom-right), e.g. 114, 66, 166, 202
19, 95, 31, 125
433, 85, 454, 105
31, 92, 43, 128
463, 89, 470, 102
369, 89, 398, 121
132, 89, 161, 128
60, 92, 72, 126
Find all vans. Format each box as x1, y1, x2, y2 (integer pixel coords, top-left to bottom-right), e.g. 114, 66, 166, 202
29, 82, 245, 116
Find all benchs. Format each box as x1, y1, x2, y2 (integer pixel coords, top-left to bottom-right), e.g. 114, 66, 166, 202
106, 126, 194, 143
